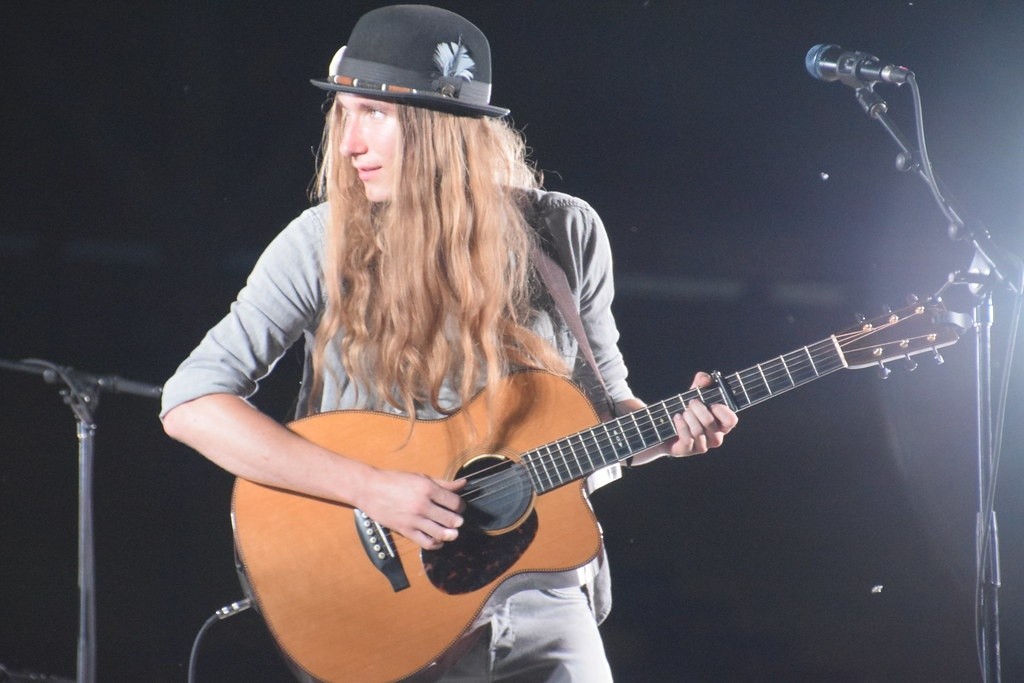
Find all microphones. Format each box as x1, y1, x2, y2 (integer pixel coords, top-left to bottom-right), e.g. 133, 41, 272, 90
804, 43, 914, 88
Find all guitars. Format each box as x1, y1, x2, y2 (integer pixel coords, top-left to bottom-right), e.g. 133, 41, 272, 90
232, 270, 982, 683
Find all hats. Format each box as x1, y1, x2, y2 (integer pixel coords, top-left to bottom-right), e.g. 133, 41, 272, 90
310, 3, 510, 118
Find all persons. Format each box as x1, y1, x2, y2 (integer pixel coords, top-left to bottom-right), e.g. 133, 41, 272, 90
159, 3, 739, 682
